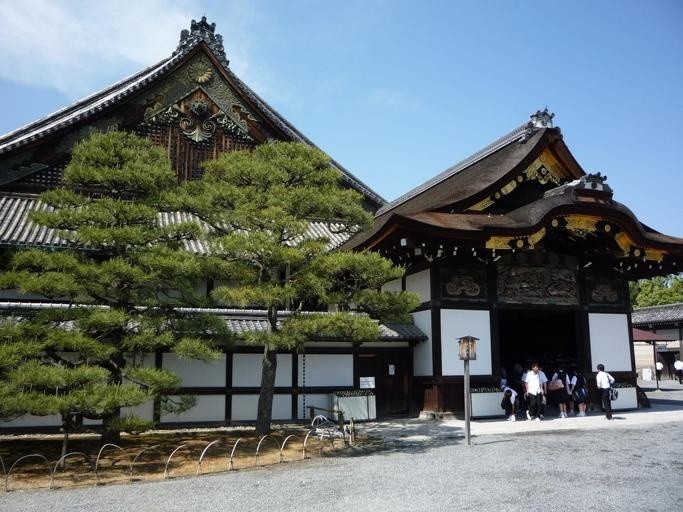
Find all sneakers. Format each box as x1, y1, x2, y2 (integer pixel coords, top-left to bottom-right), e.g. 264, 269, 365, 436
506, 412, 612, 421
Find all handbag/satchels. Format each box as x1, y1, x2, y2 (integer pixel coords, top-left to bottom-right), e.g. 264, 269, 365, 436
547, 379, 564, 390
608, 388, 617, 401
573, 387, 588, 403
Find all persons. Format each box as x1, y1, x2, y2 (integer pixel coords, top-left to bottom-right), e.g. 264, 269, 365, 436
673, 359, 683, 384
595, 364, 615, 418
494, 357, 597, 421
656, 360, 664, 380
